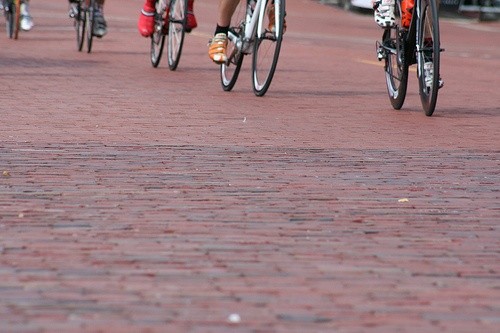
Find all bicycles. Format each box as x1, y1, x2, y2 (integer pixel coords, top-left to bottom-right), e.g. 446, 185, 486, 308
0, 0, 24, 41
147, 0, 188, 71
219, 0, 286, 96
371, 0, 444, 117
67, 0, 98, 54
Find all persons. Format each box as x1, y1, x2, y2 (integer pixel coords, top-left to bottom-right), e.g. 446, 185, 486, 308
0, 0, 33, 31
208, 0, 287, 63
374, 0, 444, 90
68, 0, 107, 38
138, 0, 197, 37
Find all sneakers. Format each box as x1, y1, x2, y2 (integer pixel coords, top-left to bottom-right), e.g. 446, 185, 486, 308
373, 0, 396, 27
69, 0, 80, 17
90, 7, 107, 35
208, 33, 228, 62
184, 0, 197, 30
138, 4, 156, 36
267, 4, 286, 35
19, 2, 35, 31
425, 62, 443, 90
